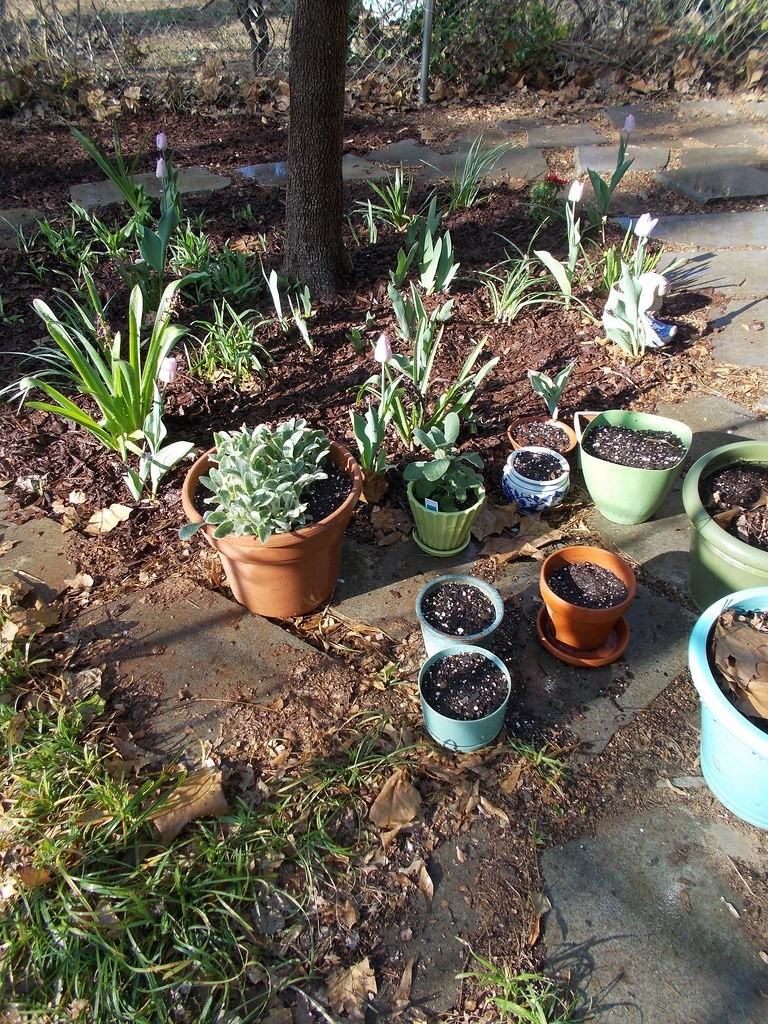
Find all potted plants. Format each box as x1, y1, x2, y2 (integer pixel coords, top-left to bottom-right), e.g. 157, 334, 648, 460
178, 416, 363, 618
406, 412, 486, 559
507, 360, 579, 455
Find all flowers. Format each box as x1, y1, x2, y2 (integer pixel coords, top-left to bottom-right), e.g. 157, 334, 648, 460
349, 331, 406, 478
588, 113, 637, 224
601, 212, 672, 352
136, 130, 184, 246
532, 177, 586, 310
4, 270, 205, 502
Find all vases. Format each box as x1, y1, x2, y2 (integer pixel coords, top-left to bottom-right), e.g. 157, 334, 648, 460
503, 448, 572, 512
688, 586, 768, 832
577, 408, 692, 526
413, 576, 504, 659
534, 545, 637, 667
683, 441, 768, 610
417, 645, 512, 754
